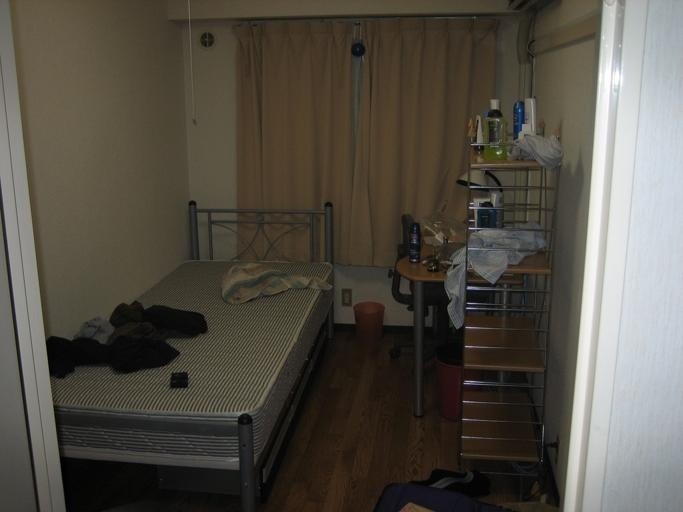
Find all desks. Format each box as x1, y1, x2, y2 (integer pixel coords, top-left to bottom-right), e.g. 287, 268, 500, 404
395, 236, 524, 418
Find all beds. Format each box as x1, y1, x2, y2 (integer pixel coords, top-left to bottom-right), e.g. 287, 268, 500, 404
48, 200, 334, 512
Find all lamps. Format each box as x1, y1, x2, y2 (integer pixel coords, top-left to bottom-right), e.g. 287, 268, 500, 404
457, 169, 504, 192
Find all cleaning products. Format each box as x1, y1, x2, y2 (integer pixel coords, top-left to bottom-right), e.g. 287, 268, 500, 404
483, 99, 507, 162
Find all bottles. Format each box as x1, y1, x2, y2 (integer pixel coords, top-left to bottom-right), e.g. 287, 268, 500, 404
488, 98, 503, 140
408, 221, 421, 264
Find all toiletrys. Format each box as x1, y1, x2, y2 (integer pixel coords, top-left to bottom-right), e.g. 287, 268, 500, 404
514, 99, 537, 138
473, 113, 485, 150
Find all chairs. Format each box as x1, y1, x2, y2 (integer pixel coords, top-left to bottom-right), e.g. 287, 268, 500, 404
392, 214, 464, 361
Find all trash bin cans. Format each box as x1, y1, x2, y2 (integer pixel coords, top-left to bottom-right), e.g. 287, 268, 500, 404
435, 340, 480, 422
354, 302, 384, 340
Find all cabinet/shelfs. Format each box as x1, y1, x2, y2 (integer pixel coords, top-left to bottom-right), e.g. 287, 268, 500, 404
459, 148, 561, 490
